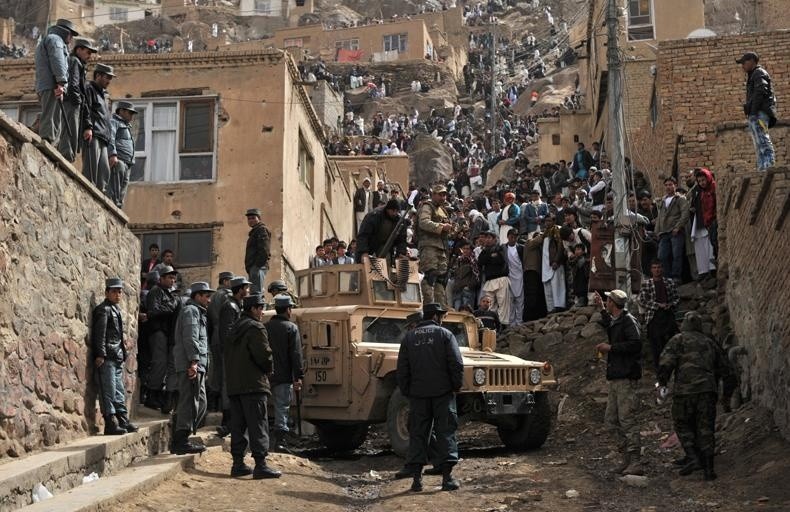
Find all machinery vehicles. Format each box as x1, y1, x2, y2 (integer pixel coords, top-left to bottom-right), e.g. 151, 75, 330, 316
261, 207, 559, 458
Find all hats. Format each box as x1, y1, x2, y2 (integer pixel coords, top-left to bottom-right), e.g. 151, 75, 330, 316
245, 209, 261, 216
681, 310, 704, 333
106, 278, 124, 288
158, 266, 174, 274
432, 184, 447, 193
57, 18, 138, 114
604, 289, 627, 306
190, 283, 217, 293
423, 303, 447, 315
219, 272, 296, 306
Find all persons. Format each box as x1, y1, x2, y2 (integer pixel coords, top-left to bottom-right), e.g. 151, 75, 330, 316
171, 281, 217, 455
227, 296, 282, 479
139, 244, 295, 415
264, 294, 303, 455
29, 18, 136, 204
295, 1, 717, 334
735, 53, 779, 172
592, 290, 643, 472
245, 208, 271, 300
658, 311, 718, 479
93, 278, 139, 434
398, 304, 464, 494
138, 22, 219, 53
1, 42, 27, 58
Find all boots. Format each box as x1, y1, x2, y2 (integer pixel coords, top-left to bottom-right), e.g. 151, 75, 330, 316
103, 415, 127, 435
673, 453, 717, 480
621, 452, 643, 476
216, 424, 230, 437
435, 296, 455, 313
608, 451, 630, 475
230, 454, 254, 477
176, 431, 206, 454
145, 388, 173, 413
169, 435, 177, 453
394, 462, 460, 491
115, 411, 138, 432
253, 457, 281, 478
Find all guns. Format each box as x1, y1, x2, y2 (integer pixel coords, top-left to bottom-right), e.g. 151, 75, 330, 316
442, 246, 462, 288
381, 204, 412, 259
